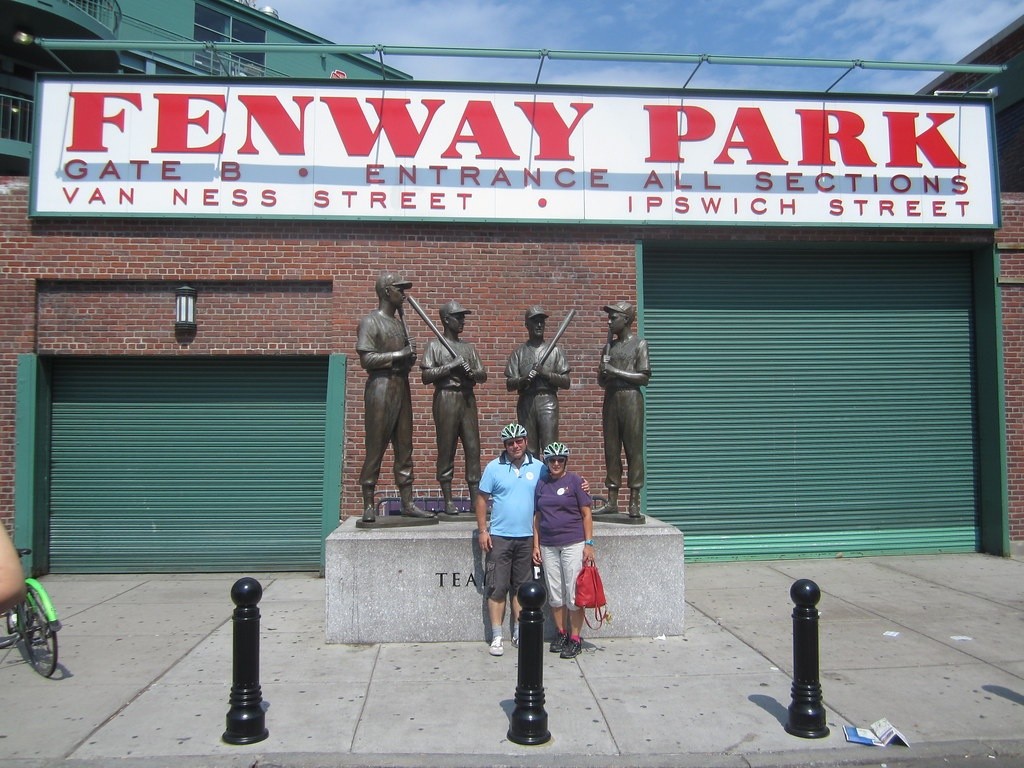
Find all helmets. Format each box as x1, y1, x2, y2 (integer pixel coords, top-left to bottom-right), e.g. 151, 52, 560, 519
544, 442, 569, 460
501, 423, 527, 442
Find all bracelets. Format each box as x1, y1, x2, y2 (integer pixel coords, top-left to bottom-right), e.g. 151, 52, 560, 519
479, 528, 488, 536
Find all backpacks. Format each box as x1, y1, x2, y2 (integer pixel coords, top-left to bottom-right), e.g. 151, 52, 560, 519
575, 558, 607, 630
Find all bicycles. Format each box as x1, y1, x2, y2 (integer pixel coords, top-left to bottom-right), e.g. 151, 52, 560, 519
0, 549, 62, 678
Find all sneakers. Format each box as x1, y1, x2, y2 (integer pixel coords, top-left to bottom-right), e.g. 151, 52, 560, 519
560, 638, 582, 658
490, 637, 504, 656
550, 633, 568, 652
511, 637, 520, 648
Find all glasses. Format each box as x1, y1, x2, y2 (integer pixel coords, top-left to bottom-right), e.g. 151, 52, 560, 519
505, 438, 525, 446
547, 458, 566, 462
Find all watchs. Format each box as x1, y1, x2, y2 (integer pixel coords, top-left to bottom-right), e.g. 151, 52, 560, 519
586, 539, 594, 546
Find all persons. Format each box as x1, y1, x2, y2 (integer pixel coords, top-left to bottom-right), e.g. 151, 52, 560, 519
504, 305, 571, 463
0, 524, 25, 614
589, 302, 651, 518
356, 273, 435, 521
419, 302, 490, 516
533, 441, 596, 658
478, 421, 588, 656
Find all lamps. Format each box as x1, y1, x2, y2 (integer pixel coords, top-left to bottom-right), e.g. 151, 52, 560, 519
175, 282, 198, 334
